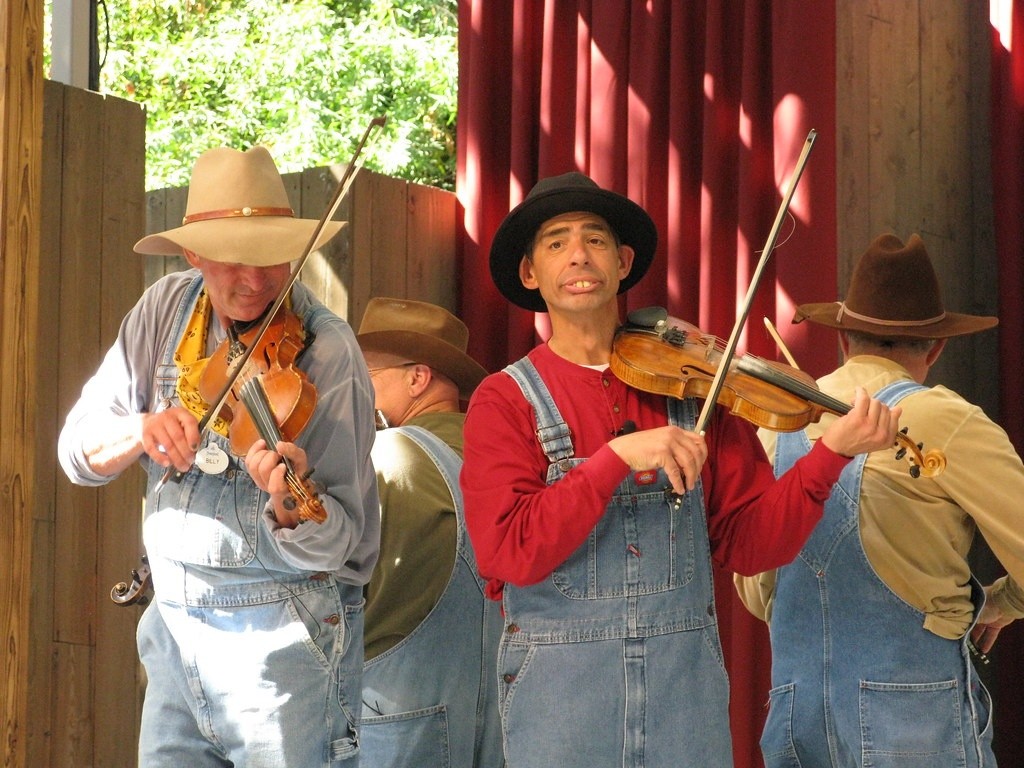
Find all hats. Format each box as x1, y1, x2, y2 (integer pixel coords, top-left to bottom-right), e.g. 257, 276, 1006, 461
489, 172, 658, 313
132, 146, 349, 268
794, 232, 999, 338
356, 297, 490, 401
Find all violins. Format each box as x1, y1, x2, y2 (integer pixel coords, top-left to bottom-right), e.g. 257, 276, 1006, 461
609, 314, 947, 479
200, 306, 328, 525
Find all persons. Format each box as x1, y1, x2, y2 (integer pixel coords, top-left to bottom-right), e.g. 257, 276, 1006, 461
52, 148, 376, 768
729, 234, 1024, 768
353, 297, 503, 767
457, 171, 902, 767
